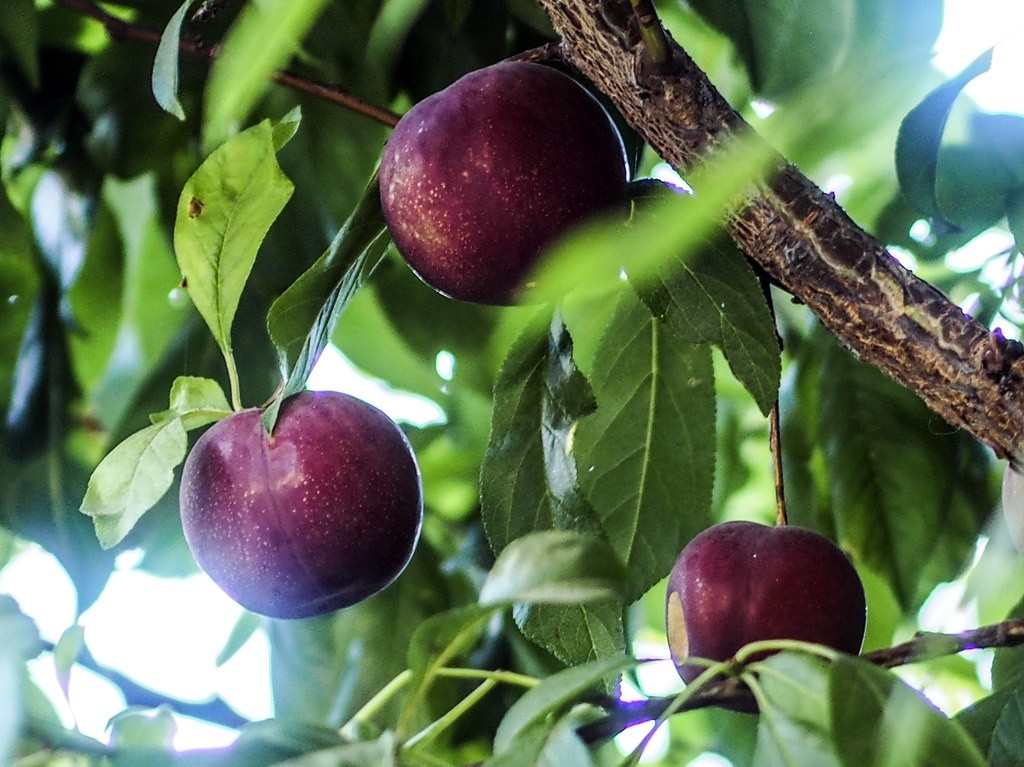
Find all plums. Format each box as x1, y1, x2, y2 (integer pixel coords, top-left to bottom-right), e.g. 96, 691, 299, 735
178, 388, 422, 623
378, 62, 629, 304
665, 522, 866, 713
